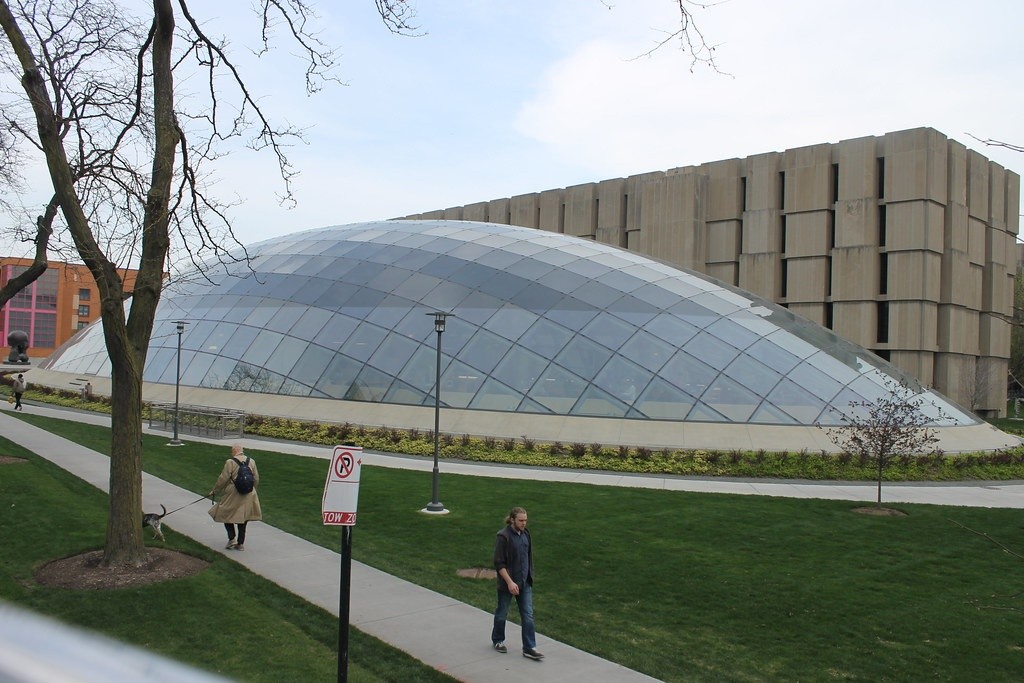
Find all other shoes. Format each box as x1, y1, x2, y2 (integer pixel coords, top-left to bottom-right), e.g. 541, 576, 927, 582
19, 407, 22, 411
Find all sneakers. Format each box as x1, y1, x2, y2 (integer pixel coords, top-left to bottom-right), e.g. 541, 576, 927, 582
494, 642, 508, 653
225, 538, 237, 548
522, 648, 544, 659
234, 544, 244, 551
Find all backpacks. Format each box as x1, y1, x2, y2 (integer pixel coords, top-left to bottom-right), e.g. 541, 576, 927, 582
8, 396, 13, 404
228, 457, 254, 496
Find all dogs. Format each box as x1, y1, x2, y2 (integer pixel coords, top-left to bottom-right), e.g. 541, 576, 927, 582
142, 504, 166, 543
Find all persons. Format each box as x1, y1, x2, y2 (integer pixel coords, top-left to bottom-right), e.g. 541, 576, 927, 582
11, 374, 25, 411
207, 443, 263, 551
491, 507, 545, 659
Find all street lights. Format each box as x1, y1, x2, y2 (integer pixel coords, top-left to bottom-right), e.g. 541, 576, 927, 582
425, 309, 457, 511
170, 320, 190, 445
